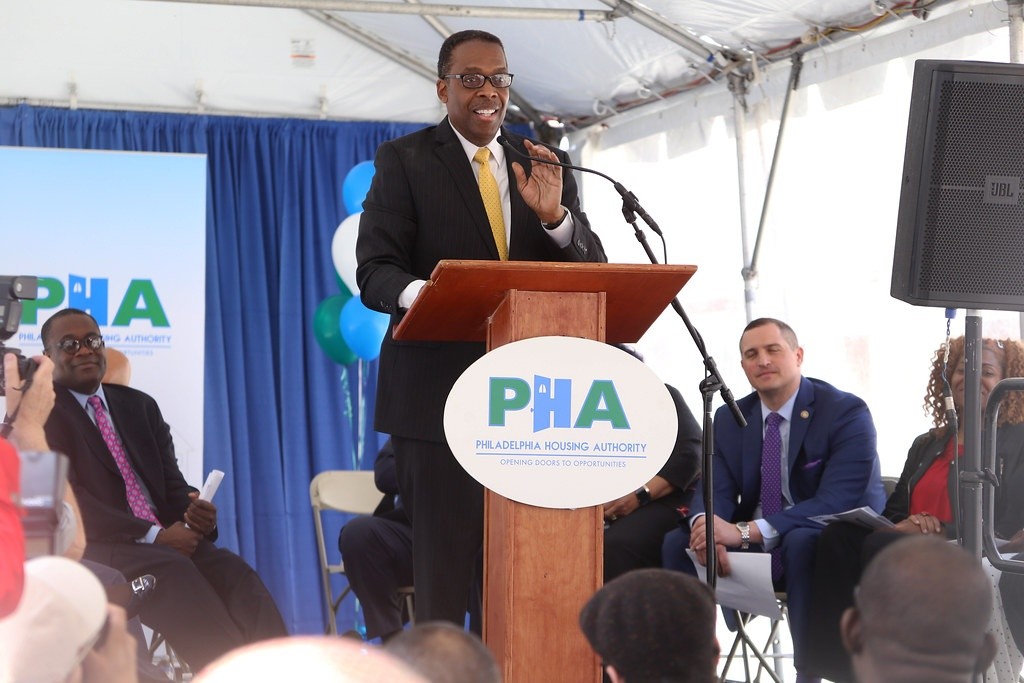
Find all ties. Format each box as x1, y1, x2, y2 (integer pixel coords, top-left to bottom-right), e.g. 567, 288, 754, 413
471, 147, 507, 263
88, 395, 159, 523
760, 412, 785, 520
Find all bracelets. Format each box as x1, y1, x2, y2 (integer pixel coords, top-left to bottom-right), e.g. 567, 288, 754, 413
643, 485, 650, 494
542, 211, 567, 230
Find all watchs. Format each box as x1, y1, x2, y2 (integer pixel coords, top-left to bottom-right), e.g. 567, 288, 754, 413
737, 522, 750, 549
636, 487, 649, 507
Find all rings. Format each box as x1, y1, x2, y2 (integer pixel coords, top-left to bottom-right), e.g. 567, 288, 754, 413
612, 515, 617, 520
924, 514, 929, 517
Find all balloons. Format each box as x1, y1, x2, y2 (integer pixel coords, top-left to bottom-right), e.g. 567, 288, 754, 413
313, 161, 390, 365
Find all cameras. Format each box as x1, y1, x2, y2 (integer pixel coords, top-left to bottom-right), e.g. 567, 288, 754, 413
0, 276, 38, 397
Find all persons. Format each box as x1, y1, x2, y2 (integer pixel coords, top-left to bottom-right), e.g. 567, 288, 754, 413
0, 353, 86, 563
579, 569, 720, 683
193, 633, 433, 683
841, 536, 997, 683
41, 308, 289, 683
806, 336, 1024, 683
661, 318, 886, 683
356, 29, 607, 634
603, 343, 702, 582
388, 624, 499, 683
0, 559, 139, 683
338, 438, 482, 644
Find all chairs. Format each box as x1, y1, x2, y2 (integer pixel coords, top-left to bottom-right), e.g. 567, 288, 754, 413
718, 474, 903, 683
308, 470, 399, 634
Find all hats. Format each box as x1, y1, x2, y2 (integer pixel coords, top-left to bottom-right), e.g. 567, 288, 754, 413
0, 555, 109, 683
578, 569, 718, 683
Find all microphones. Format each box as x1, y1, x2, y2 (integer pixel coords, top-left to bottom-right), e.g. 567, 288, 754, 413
496, 135, 663, 236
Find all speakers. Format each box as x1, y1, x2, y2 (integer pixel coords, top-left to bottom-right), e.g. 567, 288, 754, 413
891, 60, 1024, 313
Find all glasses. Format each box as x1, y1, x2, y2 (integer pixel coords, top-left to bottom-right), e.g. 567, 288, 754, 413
44, 335, 107, 353
446, 74, 514, 91
